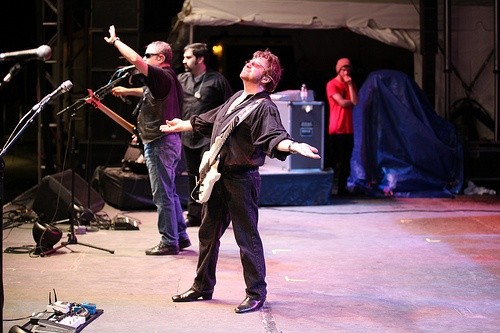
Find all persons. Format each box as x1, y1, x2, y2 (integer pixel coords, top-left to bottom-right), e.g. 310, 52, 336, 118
327, 58, 360, 200
105, 25, 191, 255
160, 50, 321, 313
178, 42, 232, 227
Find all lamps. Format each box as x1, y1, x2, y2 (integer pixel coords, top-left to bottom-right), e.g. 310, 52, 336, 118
33, 220, 62, 255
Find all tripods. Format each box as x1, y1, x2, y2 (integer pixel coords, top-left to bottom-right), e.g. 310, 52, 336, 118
40, 83, 120, 256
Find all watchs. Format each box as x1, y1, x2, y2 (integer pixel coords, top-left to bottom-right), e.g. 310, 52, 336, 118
113, 37, 120, 45
288, 141, 299, 154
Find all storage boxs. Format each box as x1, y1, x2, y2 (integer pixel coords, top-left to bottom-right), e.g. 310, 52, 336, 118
264, 100, 324, 172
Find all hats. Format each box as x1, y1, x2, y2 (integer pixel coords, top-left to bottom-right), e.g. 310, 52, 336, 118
336, 58, 351, 72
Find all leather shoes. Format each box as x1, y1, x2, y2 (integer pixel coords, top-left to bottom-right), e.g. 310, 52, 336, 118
172, 287, 213, 302
234, 297, 265, 313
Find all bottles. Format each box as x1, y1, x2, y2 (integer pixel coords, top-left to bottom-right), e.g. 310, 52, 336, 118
300, 84, 307, 101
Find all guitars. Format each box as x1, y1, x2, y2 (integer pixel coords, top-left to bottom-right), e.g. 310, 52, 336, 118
198, 115, 241, 202
84, 89, 145, 156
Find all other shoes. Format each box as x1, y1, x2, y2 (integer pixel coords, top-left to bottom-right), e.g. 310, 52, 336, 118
145, 241, 179, 255
178, 239, 192, 250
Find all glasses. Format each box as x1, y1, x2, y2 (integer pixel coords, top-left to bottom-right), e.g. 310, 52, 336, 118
144, 53, 159, 58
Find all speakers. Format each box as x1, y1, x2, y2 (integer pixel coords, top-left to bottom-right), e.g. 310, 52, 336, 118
48, 0, 141, 182
267, 100, 325, 169
38, 169, 105, 221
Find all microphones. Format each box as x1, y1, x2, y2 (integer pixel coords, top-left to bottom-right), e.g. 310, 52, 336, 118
0, 45, 52, 62
32, 80, 73, 111
